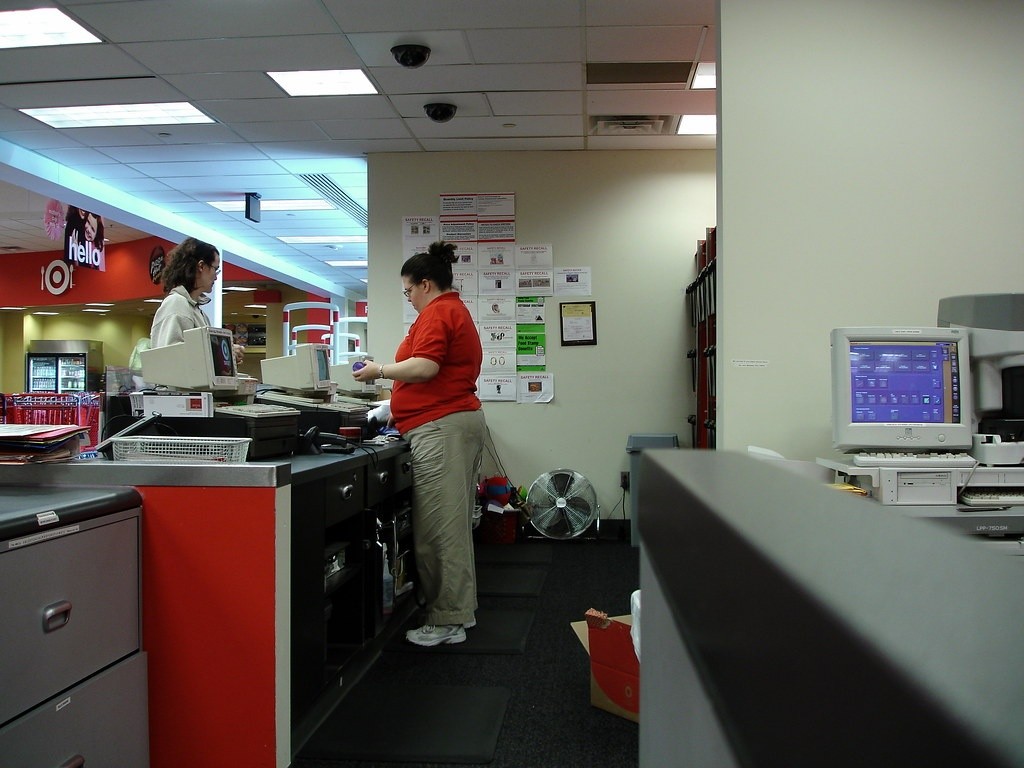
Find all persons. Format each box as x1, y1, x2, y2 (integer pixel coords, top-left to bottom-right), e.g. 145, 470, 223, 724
65, 205, 105, 271
148, 236, 245, 365
352, 240, 485, 647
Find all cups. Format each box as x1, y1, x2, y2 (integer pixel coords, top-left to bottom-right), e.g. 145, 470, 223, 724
340, 427, 361, 443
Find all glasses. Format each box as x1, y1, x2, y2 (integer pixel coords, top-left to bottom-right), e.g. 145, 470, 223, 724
404, 281, 420, 297
207, 263, 221, 276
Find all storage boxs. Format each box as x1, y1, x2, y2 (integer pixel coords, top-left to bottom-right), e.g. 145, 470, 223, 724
570, 611, 641, 722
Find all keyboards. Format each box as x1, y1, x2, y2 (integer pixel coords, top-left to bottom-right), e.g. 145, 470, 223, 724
215, 402, 302, 418
318, 403, 370, 412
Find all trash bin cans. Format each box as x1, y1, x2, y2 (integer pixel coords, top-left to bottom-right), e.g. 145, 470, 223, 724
625, 432, 679, 548
487, 509, 521, 544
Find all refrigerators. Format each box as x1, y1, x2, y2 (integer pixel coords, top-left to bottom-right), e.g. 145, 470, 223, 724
26, 340, 104, 393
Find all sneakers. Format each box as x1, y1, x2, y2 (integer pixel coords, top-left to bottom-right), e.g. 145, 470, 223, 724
463, 619, 476, 630
406, 623, 465, 646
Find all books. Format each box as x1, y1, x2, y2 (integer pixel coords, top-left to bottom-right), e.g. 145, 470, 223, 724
0, 423, 92, 465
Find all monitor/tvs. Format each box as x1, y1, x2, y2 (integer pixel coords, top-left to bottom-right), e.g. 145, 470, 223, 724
261, 343, 334, 407
140, 327, 238, 400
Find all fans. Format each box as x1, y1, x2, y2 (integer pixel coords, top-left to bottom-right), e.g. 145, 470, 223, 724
526, 468, 600, 539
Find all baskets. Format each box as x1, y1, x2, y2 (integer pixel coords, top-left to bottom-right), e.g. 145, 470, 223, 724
109, 436, 252, 464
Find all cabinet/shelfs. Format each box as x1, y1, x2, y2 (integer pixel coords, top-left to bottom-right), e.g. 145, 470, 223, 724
0, 508, 151, 768
686, 225, 717, 450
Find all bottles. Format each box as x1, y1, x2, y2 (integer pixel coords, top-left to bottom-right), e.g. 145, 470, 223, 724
33, 358, 85, 394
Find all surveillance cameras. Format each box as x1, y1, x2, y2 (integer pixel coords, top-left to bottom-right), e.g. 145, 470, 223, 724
390, 45, 431, 69
423, 103, 459, 124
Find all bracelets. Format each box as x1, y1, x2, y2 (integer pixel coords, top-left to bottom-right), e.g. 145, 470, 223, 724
379, 364, 384, 379
